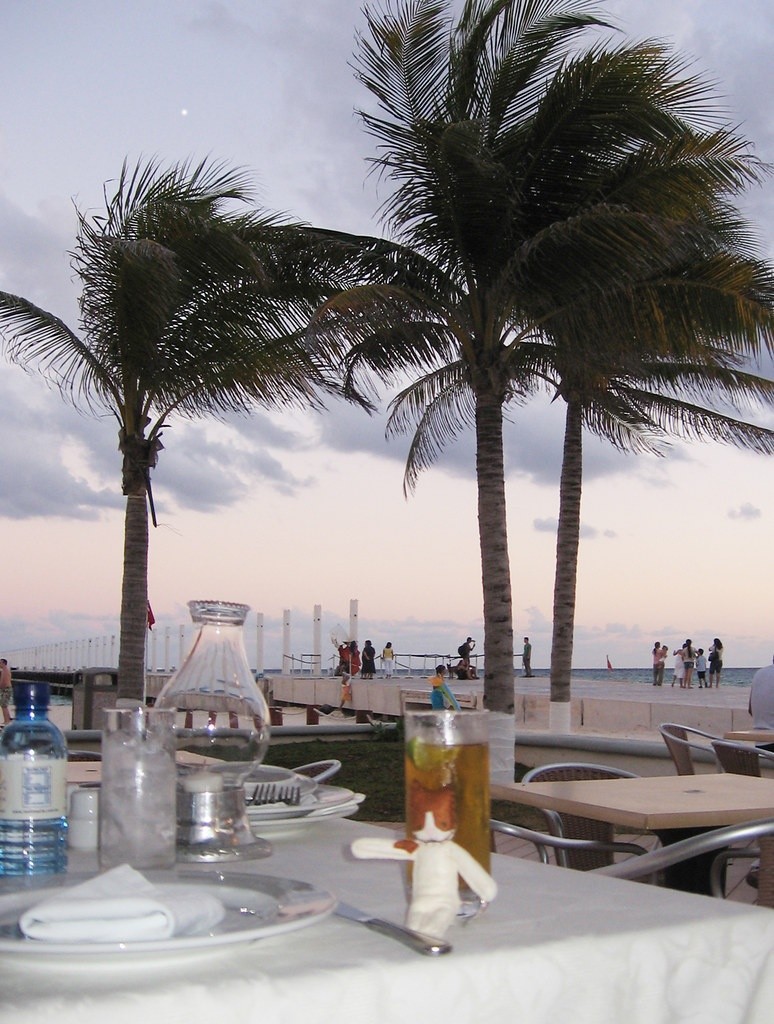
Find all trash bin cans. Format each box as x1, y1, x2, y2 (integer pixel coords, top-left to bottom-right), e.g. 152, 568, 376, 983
71, 667, 121, 730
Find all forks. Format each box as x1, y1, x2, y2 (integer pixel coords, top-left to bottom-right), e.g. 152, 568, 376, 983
277, 786, 302, 805
249, 783, 277, 807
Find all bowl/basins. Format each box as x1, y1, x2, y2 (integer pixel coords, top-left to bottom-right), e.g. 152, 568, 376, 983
68, 819, 98, 850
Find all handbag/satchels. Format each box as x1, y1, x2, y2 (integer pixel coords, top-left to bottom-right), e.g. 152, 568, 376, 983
708, 651, 718, 662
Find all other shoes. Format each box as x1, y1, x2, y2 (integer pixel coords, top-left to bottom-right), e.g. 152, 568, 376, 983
699, 686, 702, 688
653, 683, 661, 686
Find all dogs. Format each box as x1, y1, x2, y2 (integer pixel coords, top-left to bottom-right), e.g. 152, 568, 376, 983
446, 663, 457, 680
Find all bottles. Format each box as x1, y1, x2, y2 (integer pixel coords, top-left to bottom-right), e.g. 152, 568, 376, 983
0, 679, 68, 880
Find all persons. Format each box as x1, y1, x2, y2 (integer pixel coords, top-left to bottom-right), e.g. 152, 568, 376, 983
432, 665, 454, 710
652, 638, 724, 689
523, 637, 531, 677
0, 659, 12, 725
360, 640, 375, 679
383, 642, 393, 679
748, 654, 774, 753
458, 637, 480, 680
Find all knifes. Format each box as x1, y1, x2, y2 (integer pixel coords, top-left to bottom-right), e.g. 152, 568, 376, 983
333, 901, 452, 955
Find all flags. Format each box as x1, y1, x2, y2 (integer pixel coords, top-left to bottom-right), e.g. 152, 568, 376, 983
148, 599, 155, 631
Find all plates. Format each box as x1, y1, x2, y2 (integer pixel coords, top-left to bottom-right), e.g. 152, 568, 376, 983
0, 869, 336, 958
177, 763, 365, 829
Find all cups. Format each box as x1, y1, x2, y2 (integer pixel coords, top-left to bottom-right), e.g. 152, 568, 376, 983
404, 708, 491, 902
98, 706, 177, 869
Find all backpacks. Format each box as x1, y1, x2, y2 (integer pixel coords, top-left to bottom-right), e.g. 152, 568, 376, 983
458, 645, 464, 655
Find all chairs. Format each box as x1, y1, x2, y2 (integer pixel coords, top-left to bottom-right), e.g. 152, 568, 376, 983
291, 759, 344, 783
486, 722, 774, 911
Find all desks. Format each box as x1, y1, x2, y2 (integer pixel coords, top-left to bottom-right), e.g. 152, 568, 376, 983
484, 773, 774, 899
0, 762, 774, 1024
